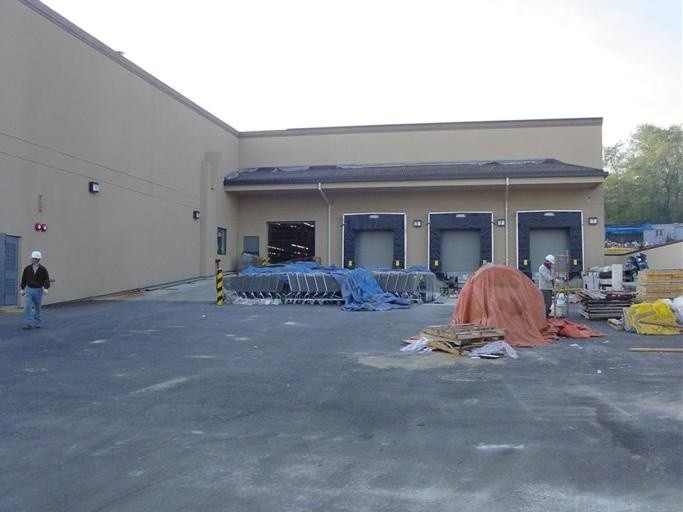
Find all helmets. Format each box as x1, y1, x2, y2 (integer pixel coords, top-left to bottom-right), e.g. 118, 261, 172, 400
29, 251, 42, 259
545, 254, 555, 264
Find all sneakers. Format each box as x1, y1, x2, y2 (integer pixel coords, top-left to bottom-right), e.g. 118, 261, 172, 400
22, 322, 42, 329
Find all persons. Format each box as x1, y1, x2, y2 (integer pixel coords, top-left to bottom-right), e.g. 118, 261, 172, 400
18, 250, 49, 330
538, 253, 556, 318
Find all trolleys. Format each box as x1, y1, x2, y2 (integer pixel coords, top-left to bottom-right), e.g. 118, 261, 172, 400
223, 270, 424, 306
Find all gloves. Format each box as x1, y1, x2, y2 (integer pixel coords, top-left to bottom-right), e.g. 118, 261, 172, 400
43, 289, 48, 297
20, 289, 25, 296
